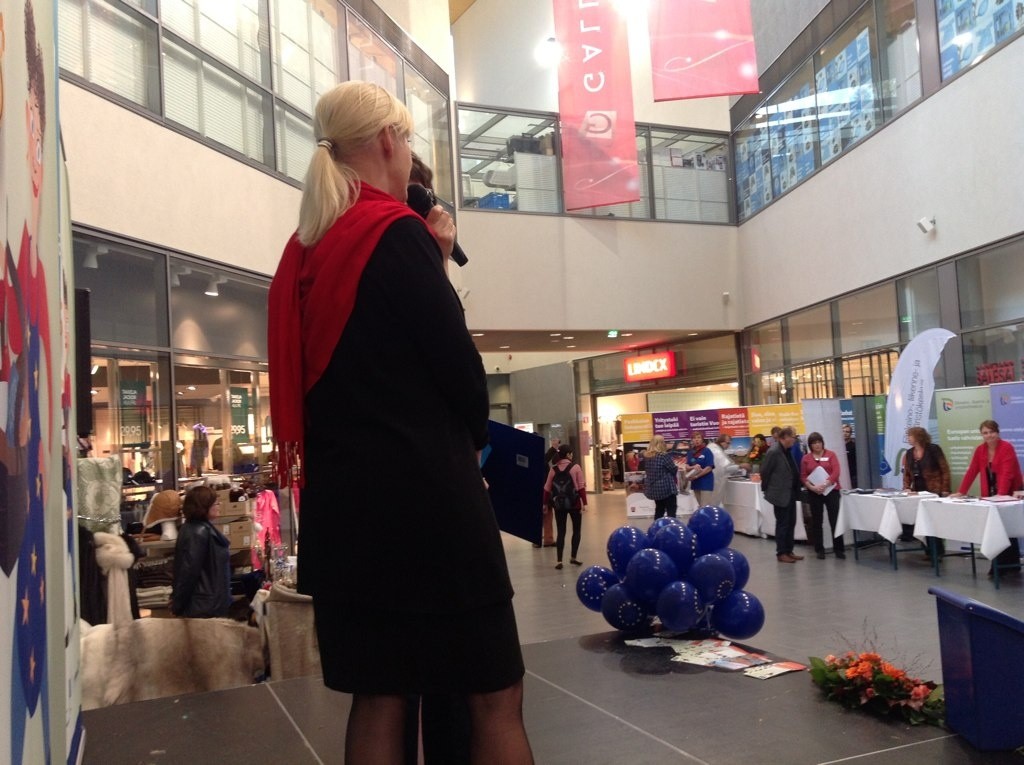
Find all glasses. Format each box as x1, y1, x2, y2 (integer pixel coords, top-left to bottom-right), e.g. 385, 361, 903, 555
725, 441, 730, 444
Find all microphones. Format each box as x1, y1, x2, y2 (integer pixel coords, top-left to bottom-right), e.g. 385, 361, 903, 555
405, 183, 469, 268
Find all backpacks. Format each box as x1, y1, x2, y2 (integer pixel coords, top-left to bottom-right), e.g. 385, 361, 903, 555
549, 463, 579, 509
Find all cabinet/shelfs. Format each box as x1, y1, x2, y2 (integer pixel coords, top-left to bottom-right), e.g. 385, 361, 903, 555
136, 541, 178, 609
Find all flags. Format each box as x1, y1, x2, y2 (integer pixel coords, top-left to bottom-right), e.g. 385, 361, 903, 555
881, 327, 958, 490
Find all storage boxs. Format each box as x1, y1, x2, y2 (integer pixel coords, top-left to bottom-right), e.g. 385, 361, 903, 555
208, 488, 257, 573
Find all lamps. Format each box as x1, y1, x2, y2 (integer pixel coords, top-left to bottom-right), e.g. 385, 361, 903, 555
81, 245, 108, 269
918, 217, 936, 234
203, 276, 229, 296
780, 385, 787, 395
170, 265, 192, 288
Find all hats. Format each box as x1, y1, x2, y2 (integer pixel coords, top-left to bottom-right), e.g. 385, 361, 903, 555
142, 489, 181, 532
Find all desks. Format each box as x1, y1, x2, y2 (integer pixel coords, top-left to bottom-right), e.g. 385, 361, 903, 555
833, 491, 940, 571
720, 479, 808, 541
912, 496, 1024, 589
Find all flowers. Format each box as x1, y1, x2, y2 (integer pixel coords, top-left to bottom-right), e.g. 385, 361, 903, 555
807, 613, 946, 727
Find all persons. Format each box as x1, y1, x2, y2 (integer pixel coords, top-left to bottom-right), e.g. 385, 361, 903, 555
902, 427, 952, 563
169, 488, 232, 618
948, 420, 1023, 575
532, 432, 556, 548
686, 431, 715, 508
627, 450, 646, 471
644, 434, 678, 520
741, 425, 857, 563
543, 444, 587, 570
267, 80, 534, 764
545, 437, 559, 475
706, 434, 752, 507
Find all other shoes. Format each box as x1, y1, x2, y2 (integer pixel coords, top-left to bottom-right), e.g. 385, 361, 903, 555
836, 553, 845, 558
789, 552, 803, 560
544, 542, 556, 547
570, 560, 583, 565
817, 550, 825, 559
777, 554, 795, 563
555, 564, 562, 569
532, 543, 540, 547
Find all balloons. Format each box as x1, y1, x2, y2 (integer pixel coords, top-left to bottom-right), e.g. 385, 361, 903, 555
652, 504, 750, 604
607, 525, 652, 581
647, 517, 680, 543
602, 584, 648, 630
625, 548, 679, 614
655, 582, 765, 640
576, 565, 619, 612
